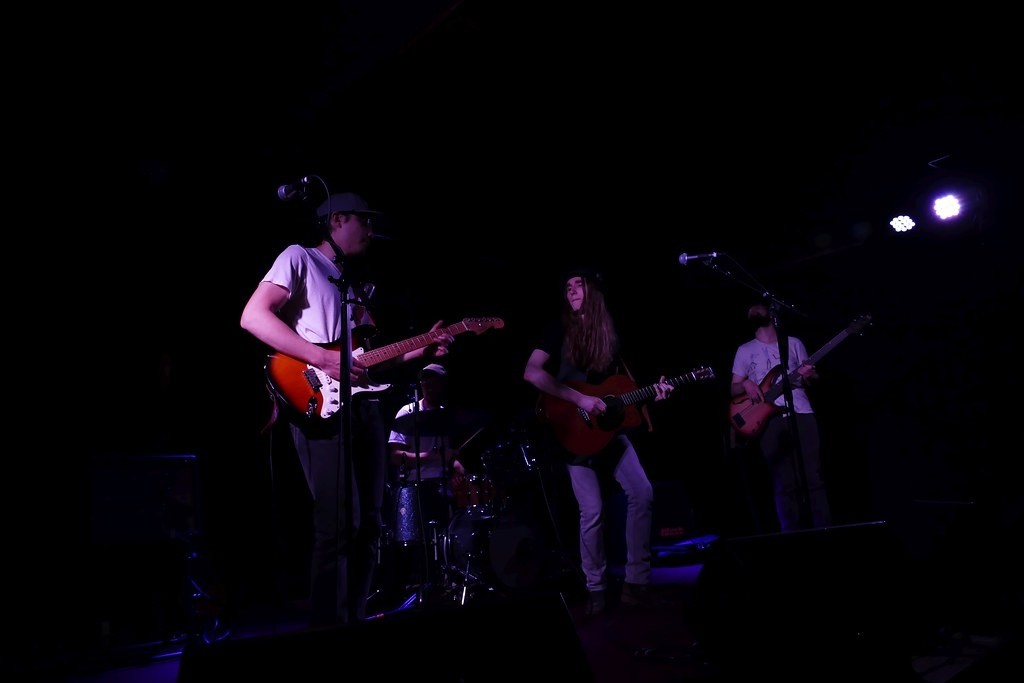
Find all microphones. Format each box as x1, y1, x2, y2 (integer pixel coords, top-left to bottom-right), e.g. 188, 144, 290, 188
679, 252, 719, 264
278, 175, 312, 200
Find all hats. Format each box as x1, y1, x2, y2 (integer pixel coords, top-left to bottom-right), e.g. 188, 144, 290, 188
316, 192, 391, 218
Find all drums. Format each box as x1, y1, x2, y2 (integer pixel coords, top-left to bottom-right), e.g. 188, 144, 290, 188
388, 482, 425, 548
445, 511, 534, 592
451, 474, 511, 510
482, 439, 550, 475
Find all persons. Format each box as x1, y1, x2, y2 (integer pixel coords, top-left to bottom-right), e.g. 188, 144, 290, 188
523, 265, 675, 625
730, 304, 831, 531
384, 362, 466, 512
237, 192, 454, 623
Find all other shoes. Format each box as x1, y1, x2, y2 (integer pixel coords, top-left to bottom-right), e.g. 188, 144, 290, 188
583, 590, 605, 621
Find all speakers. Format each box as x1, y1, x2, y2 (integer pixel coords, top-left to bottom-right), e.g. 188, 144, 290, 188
684, 519, 923, 683
176, 580, 593, 682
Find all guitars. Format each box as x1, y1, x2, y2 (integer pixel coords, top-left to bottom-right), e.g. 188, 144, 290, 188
729, 315, 874, 438
264, 316, 504, 424
545, 366, 715, 456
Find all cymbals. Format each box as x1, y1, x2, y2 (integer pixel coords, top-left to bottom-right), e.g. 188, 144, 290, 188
390, 408, 487, 435
